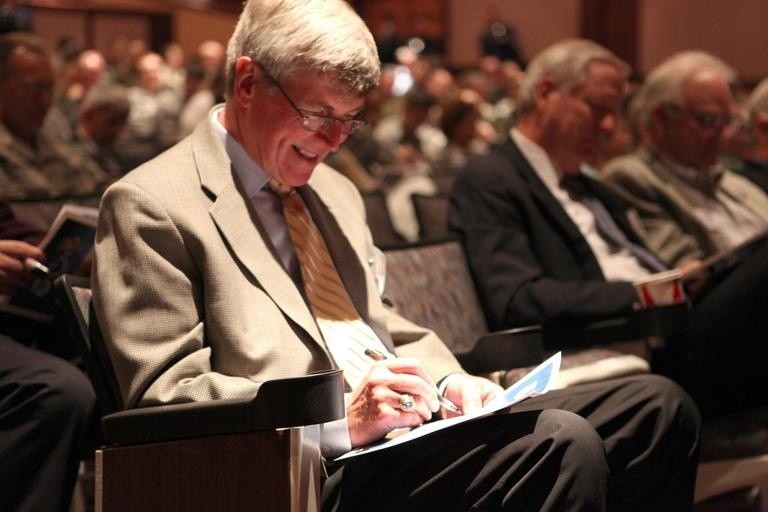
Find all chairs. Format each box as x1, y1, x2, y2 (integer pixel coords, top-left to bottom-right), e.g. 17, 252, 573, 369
10, 174, 628, 512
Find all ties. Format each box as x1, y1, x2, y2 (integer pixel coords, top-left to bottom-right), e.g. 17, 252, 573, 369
269, 177, 411, 439
560, 174, 669, 273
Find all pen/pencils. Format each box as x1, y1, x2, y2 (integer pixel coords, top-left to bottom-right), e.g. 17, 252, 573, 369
364, 348, 463, 415
23, 258, 51, 275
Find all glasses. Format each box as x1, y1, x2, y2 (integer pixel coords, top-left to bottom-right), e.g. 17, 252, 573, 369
258, 62, 368, 134
